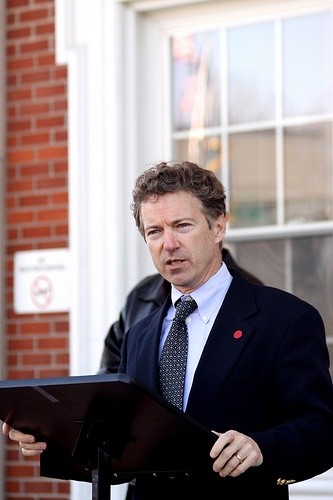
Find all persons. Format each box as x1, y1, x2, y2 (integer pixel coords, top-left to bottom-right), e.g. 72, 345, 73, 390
2, 160, 333, 500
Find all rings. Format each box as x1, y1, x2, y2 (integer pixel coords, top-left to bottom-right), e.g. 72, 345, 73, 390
235, 454, 243, 463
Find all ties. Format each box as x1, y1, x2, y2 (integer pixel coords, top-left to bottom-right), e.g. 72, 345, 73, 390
159, 295, 198, 412
